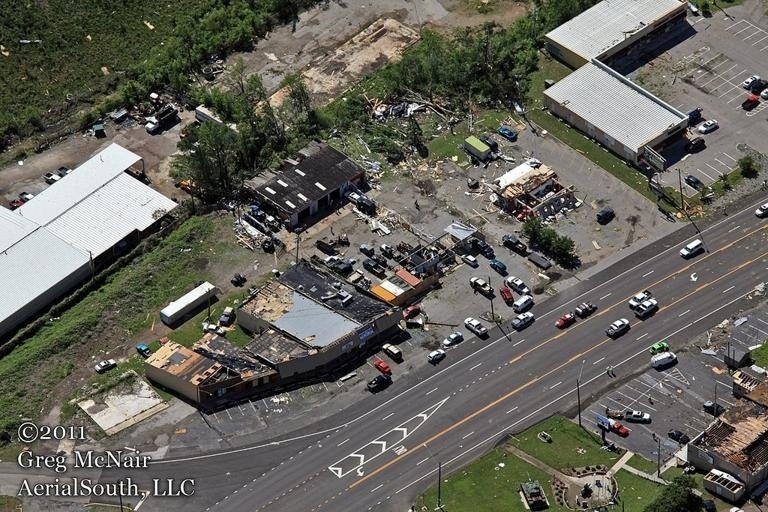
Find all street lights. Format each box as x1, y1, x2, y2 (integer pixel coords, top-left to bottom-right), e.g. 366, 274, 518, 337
576, 358, 588, 425
422, 441, 444, 509
674, 167, 685, 211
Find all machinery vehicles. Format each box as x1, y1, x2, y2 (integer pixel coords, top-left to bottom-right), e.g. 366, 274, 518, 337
519, 469, 551, 511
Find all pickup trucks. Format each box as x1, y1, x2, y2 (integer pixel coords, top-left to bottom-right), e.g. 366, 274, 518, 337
555, 290, 660, 337
8, 165, 73, 211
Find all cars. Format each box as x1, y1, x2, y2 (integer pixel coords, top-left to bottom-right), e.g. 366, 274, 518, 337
135, 343, 153, 359
220, 197, 280, 232
666, 429, 691, 447
684, 173, 705, 193
498, 127, 517, 142
596, 206, 614, 223
687, 137, 705, 155
742, 73, 768, 114
309, 230, 537, 398
648, 340, 670, 356
699, 119, 719, 134
704, 400, 725, 417
345, 190, 361, 205
94, 358, 118, 374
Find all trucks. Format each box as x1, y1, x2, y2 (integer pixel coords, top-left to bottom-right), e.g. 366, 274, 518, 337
680, 239, 703, 258
144, 103, 179, 135
219, 305, 235, 326
755, 202, 768, 219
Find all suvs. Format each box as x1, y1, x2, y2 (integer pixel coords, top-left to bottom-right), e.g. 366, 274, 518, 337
480, 135, 499, 151
649, 351, 678, 369
599, 408, 652, 437
355, 197, 376, 215
